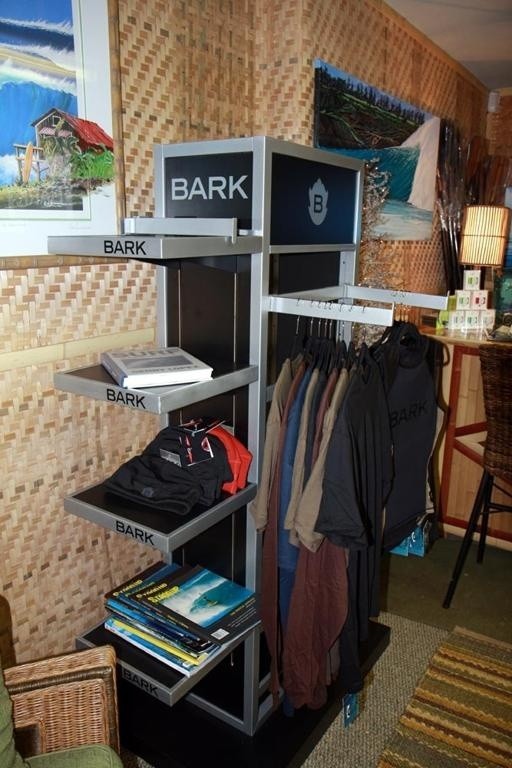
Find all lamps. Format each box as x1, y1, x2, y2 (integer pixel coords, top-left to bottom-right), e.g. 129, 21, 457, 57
454, 202, 510, 271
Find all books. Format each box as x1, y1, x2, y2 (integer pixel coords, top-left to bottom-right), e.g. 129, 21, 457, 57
101, 344, 213, 389
103, 560, 263, 680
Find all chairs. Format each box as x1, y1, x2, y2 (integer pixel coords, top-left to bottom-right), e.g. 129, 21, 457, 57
438, 343, 512, 617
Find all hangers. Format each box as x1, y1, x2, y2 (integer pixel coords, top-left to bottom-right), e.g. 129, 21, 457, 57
286, 276, 434, 383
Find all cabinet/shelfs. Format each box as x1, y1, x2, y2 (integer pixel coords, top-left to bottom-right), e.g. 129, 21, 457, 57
45, 132, 446, 736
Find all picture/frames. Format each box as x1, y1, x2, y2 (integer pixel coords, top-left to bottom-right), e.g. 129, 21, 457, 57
0, 0, 123, 259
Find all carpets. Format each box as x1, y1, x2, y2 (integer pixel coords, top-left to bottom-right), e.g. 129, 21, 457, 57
379, 625, 511, 767
